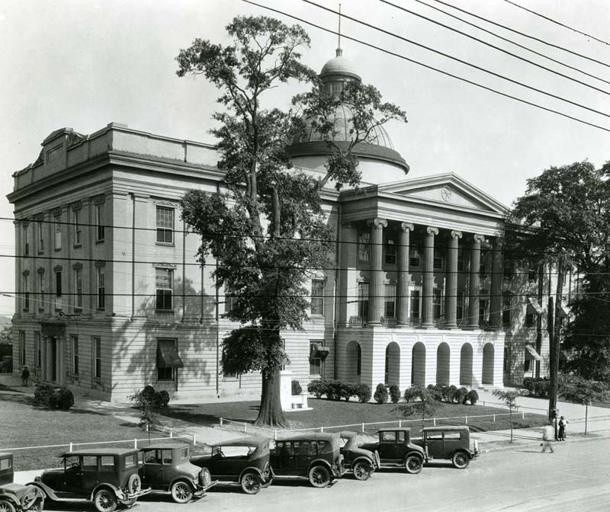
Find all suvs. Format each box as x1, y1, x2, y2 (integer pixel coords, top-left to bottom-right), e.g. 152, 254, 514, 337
34, 382, 75, 413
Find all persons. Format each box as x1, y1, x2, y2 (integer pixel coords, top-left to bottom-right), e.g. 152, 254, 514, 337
558, 416, 567, 441
543, 422, 554, 453
21, 365, 29, 385
